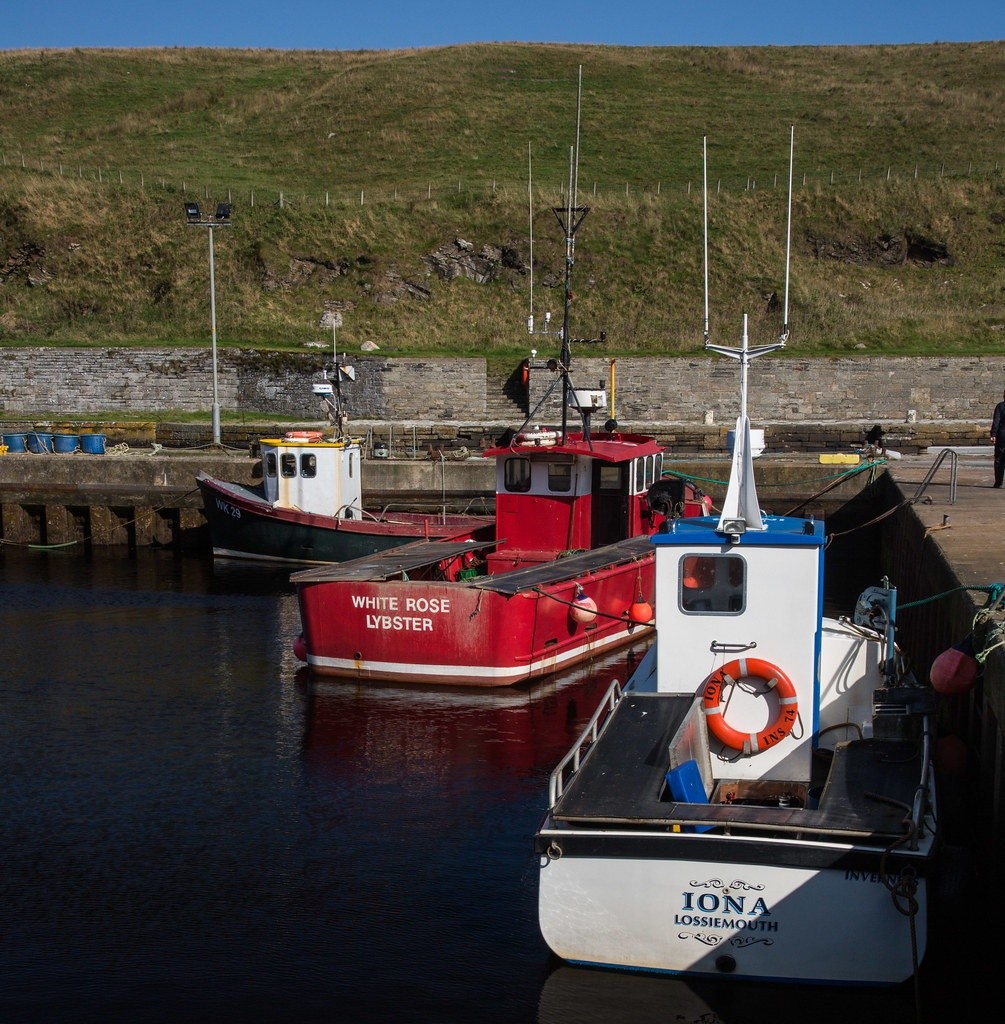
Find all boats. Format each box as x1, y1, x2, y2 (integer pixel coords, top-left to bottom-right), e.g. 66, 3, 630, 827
193, 318, 497, 576
291, 57, 713, 689
531, 121, 939, 991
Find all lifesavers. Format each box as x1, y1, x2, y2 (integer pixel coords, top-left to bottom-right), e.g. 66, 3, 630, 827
280, 428, 327, 445
696, 654, 803, 759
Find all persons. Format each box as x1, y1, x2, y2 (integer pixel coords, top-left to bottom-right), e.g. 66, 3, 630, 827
990, 391, 1005, 488
